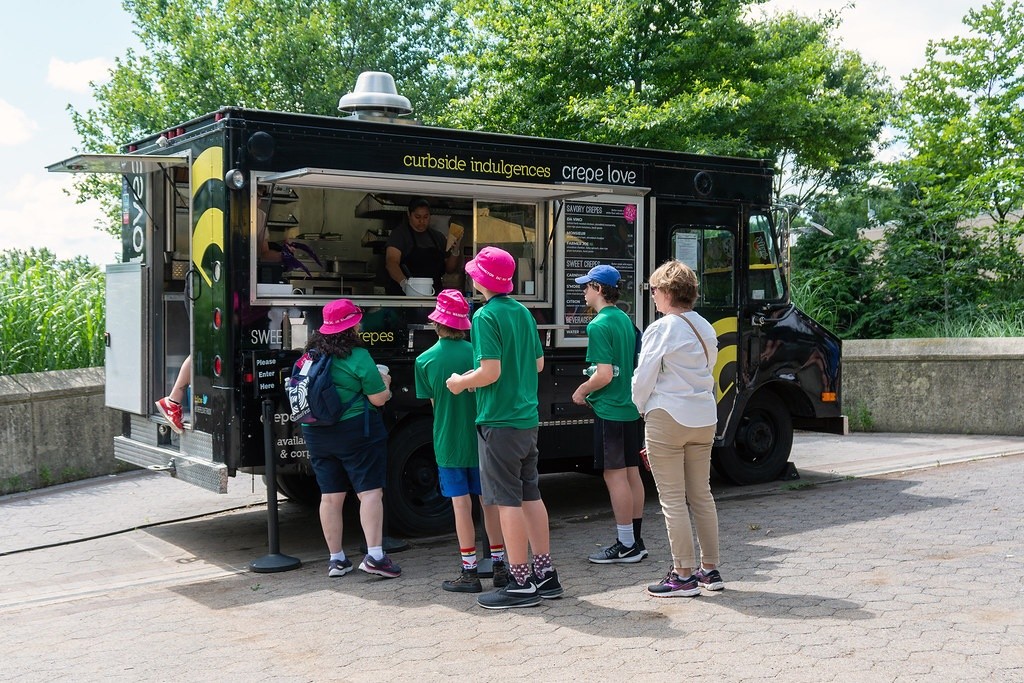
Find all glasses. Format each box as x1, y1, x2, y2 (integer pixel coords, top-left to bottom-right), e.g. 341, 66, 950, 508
651, 287, 659, 294
344, 305, 365, 319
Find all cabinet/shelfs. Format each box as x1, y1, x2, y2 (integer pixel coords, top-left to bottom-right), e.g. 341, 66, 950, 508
257, 188, 300, 227
355, 192, 473, 254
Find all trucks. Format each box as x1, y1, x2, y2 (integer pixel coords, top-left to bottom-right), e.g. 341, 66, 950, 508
41, 72, 850, 572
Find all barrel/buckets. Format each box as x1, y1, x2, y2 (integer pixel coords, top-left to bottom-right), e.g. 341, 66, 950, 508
405, 277, 436, 296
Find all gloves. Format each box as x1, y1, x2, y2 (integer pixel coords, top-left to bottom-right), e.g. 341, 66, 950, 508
400, 279, 407, 294
452, 236, 462, 256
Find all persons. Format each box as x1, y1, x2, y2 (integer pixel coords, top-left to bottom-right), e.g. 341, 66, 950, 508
571, 264, 649, 565
154, 184, 270, 435
414, 288, 513, 594
303, 298, 403, 579
631, 258, 725, 599
385, 196, 464, 297
445, 246, 565, 610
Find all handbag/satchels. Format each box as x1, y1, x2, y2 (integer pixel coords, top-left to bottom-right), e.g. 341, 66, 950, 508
632, 321, 642, 372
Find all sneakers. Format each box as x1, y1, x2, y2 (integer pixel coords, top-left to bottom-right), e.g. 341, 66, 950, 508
648, 565, 701, 597
328, 555, 354, 578
154, 397, 184, 435
530, 563, 564, 598
588, 538, 648, 563
692, 564, 724, 590
477, 574, 542, 609
358, 550, 402, 577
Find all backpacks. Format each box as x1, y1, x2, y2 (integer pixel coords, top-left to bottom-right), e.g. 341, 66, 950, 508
285, 348, 369, 438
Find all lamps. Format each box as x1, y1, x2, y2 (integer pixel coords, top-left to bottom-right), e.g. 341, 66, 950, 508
225, 146, 246, 190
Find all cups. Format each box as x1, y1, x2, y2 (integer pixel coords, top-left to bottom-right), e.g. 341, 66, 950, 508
376, 364, 390, 375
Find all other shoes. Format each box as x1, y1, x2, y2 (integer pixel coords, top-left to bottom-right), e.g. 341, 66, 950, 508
779, 373, 796, 380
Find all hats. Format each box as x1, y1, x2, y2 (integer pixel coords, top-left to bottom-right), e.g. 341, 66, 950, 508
575, 265, 621, 288
427, 289, 471, 330
319, 299, 363, 334
465, 246, 515, 293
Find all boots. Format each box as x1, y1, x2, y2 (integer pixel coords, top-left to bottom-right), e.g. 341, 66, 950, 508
442, 565, 482, 593
492, 560, 509, 587
